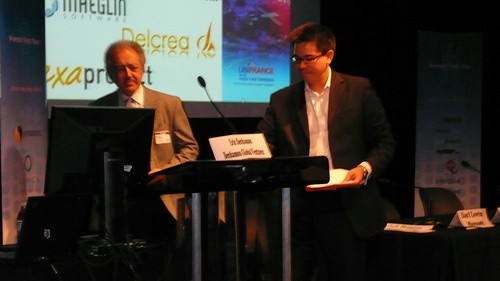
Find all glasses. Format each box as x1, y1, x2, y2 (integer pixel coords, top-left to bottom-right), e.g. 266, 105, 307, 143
290, 49, 330, 65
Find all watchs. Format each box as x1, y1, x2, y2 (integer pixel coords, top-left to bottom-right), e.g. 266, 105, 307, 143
360, 165, 368, 179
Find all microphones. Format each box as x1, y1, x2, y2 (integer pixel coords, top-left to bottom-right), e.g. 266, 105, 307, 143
461, 160, 483, 175
197, 76, 238, 134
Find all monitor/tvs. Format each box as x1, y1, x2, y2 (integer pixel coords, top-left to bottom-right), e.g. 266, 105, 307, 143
43, 105, 157, 248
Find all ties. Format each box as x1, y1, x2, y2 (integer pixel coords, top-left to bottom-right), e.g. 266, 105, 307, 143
126, 97, 133, 108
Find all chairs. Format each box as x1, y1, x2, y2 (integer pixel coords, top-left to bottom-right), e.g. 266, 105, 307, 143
380, 198, 402, 223
418, 188, 462, 217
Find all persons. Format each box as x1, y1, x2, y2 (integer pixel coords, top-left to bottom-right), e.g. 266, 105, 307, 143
255, 21, 396, 281
88, 40, 199, 222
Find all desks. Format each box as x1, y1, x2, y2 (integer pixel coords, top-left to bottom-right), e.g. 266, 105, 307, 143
377, 210, 500, 281
